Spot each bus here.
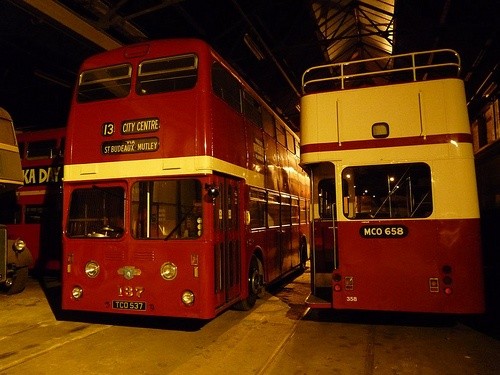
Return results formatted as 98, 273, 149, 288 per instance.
297, 49, 487, 315
1, 128, 63, 274
61, 37, 311, 321
